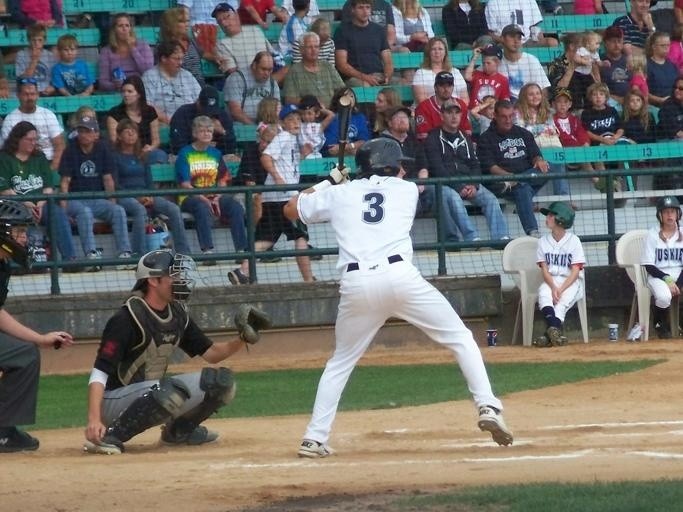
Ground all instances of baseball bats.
[338,96,352,171]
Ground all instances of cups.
[485,329,499,347]
[607,323,620,342]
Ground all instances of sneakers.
[65,248,322,286]
[537,326,567,346]
[0,426,40,454]
[81,436,125,455]
[479,405,513,446]
[160,426,219,447]
[296,439,335,458]
[654,322,671,338]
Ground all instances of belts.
[346,254,403,272]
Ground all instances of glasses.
[18,78,37,84]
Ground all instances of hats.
[200,87,221,115]
[211,3,235,18]
[72,115,99,130]
[386,25,572,119]
[278,95,317,120]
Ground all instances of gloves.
[327,162,352,185]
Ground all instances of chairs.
[615,229,683,342]
[0,0,683,235]
[502,236,589,347]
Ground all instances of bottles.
[256,122,276,140]
[111,67,127,80]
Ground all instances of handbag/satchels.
[27,234,47,267]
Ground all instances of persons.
[0,0,683,284]
[282,138,514,458]
[83,249,272,455]
[640,194,683,339]
[534,202,587,347]
[0,198,75,454]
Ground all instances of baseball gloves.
[234,303,273,343]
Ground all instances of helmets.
[135,248,197,314]
[0,200,36,271]
[539,200,574,229]
[355,138,415,178]
[656,196,682,221]
